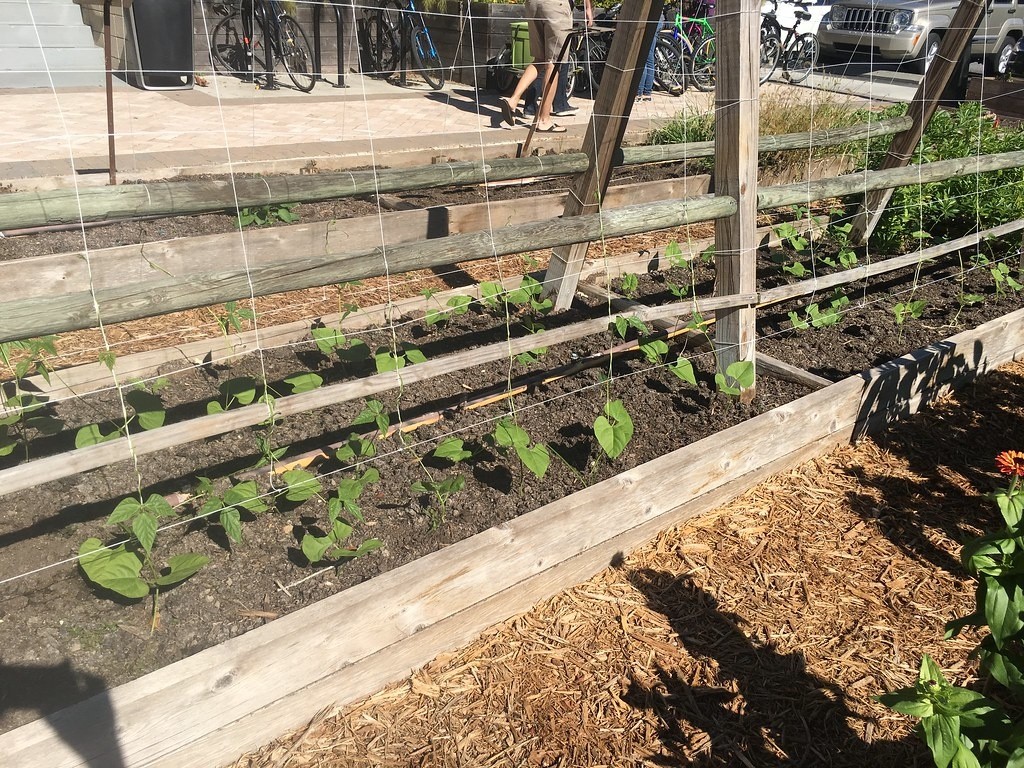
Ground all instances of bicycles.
[495,0,822,100]
[212,0,318,92]
[361,0,446,89]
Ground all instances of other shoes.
[523,103,538,114]
[635,95,644,102]
[556,106,580,115]
[643,96,651,101]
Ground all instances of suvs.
[815,0,1024,74]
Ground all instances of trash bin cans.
[511,23,534,74]
[942,42,972,105]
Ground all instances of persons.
[634,10,665,102]
[502,0,593,133]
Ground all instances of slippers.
[497,97,516,126]
[536,123,567,132]
